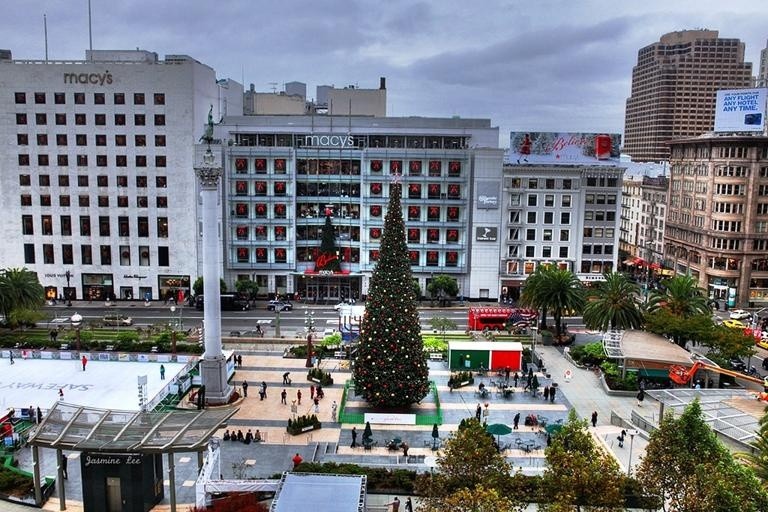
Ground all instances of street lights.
[66,270,72,306]
[169,305,176,327]
[626,429,639,476]
[304,311,316,367]
[530,327,538,363]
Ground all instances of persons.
[292,452,303,468]
[639,275,646,282]
[242,372,337,413]
[256,323,261,333]
[24,406,34,422]
[591,411,598,427]
[10,350,14,364]
[82,356,87,371]
[49,329,58,342]
[617,430,626,448]
[639,378,714,389]
[351,427,357,448]
[160,364,165,380]
[479,382,484,390]
[384,497,413,512]
[234,354,242,367]
[223,429,261,440]
[62,454,68,480]
[505,365,556,403]
[476,403,481,423]
[513,413,537,429]
[399,442,409,456]
[637,390,644,407]
[58,388,64,401]
[51,294,63,304]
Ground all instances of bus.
[468,306,540,331]
[196,294,250,311]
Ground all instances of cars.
[722,320,746,329]
[731,359,746,371]
[102,314,133,326]
[333,302,345,311]
[730,309,752,320]
[323,329,333,336]
[756,339,768,350]
[267,300,293,311]
